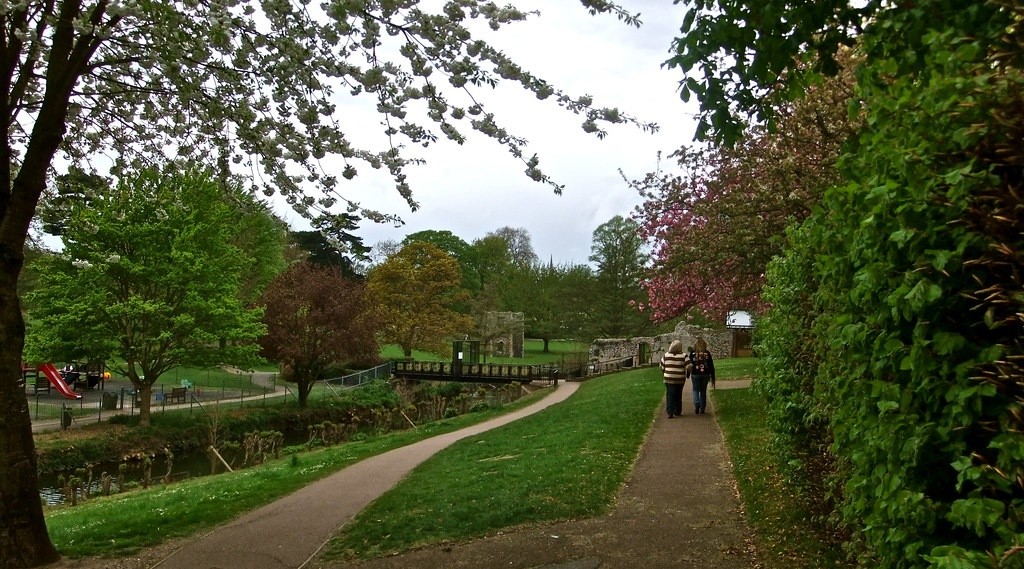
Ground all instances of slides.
[37,362,82,400]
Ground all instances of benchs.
[164,387,186,404]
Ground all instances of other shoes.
[668,413,673,419]
[700,410,704,414]
[695,404,700,414]
[674,411,681,416]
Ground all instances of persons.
[63,362,73,377]
[660,340,693,419]
[686,337,716,415]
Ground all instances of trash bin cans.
[102,392,118,410]
[63,406,72,427]
